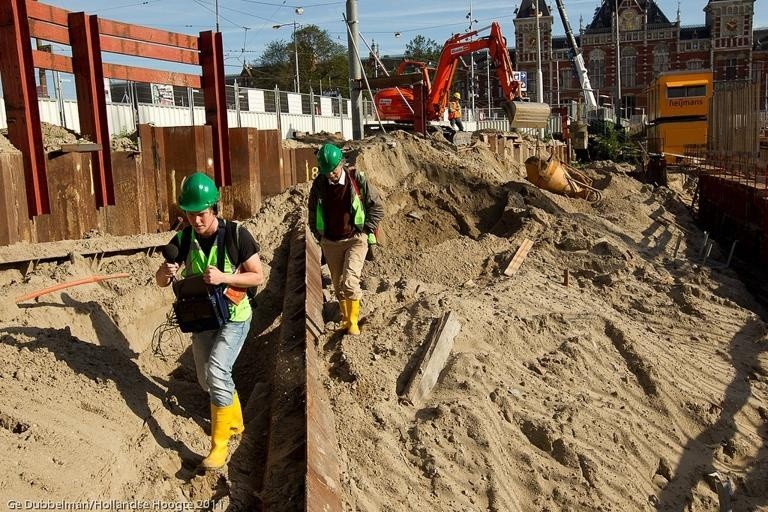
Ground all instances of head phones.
[214,201,220,213]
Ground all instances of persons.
[308,143,385,336]
[155,172,265,472]
[448,92,464,131]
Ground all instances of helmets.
[178,171,220,212]
[316,143,343,174]
[453,92,461,99]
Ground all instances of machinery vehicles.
[364,22,551,143]
[555,0,596,107]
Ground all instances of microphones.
[162,244,179,282]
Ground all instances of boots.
[200,402,234,471]
[228,389,246,439]
[338,300,349,329]
[346,299,361,335]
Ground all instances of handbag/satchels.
[172,273,232,334]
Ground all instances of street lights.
[371,40,378,78]
[272,20,300,95]
